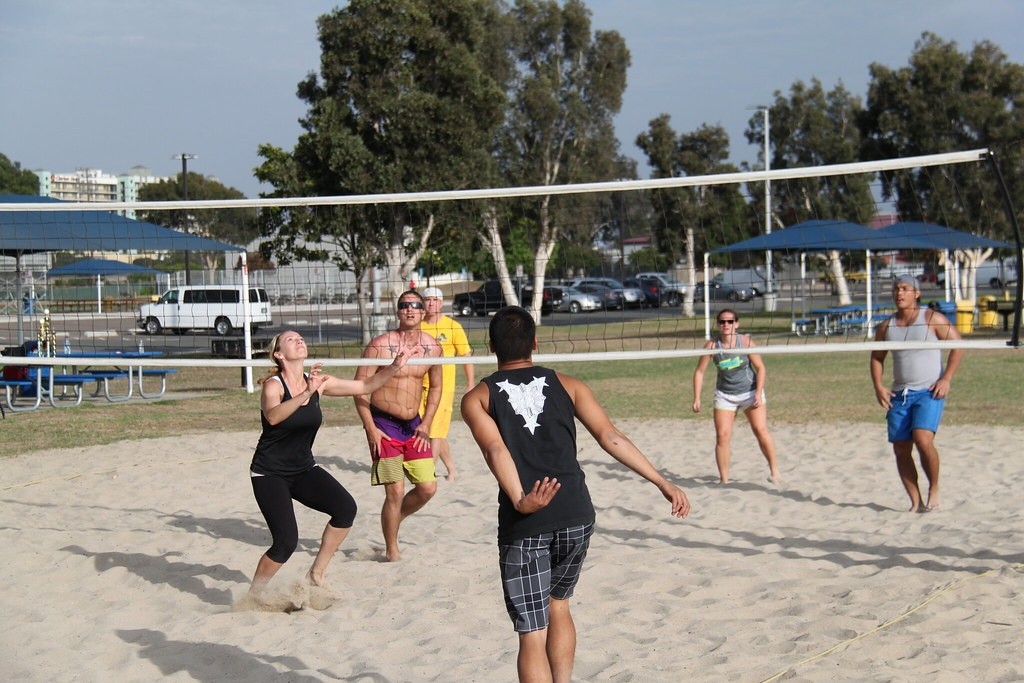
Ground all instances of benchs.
[795,315,891,337]
[0,369,179,412]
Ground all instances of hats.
[894,274,921,303]
[423,287,442,297]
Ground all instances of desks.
[27,350,162,399]
[808,303,893,335]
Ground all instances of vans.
[137,285,273,337]
[713,269,777,296]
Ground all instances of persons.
[693,309,779,483]
[354,291,443,562]
[870,274,965,513]
[460,304,690,683]
[410,280,415,291]
[418,287,474,482]
[247,331,419,594]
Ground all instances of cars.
[636,273,699,306]
[698,279,756,302]
[937,257,1018,290]
[573,285,626,307]
[624,278,661,308]
[818,264,867,282]
[543,286,602,313]
[563,278,645,309]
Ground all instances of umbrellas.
[710,219,934,332]
[870,222,1014,303]
[0,193,247,347]
[46,260,161,315]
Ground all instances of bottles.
[139,340,144,353]
[64,336,71,354]
[1004,287,1010,301]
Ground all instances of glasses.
[398,301,423,309]
[717,320,734,324]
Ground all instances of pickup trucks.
[452,280,563,317]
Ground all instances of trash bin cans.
[978,295,998,328]
[928,301,976,335]
[19,340,50,397]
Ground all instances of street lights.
[172,153,199,286]
[746,105,777,312]
